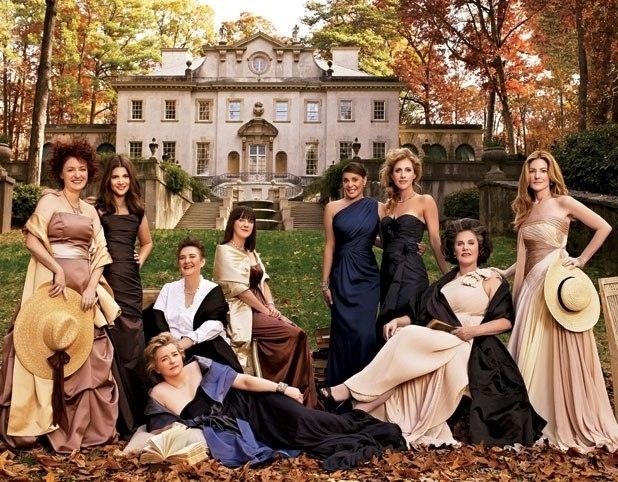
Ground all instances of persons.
[315,218,548,448]
[142,237,245,374]
[213,207,325,410]
[144,331,407,471]
[41,155,154,437]
[380,149,448,316]
[0,140,122,454]
[490,151,618,455]
[322,162,427,387]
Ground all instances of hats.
[543,259,601,332]
[13,285,95,380]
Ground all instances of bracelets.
[322,281,329,290]
[275,382,289,394]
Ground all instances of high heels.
[318,386,350,411]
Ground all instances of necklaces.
[61,190,83,216]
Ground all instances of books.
[140,424,208,467]
